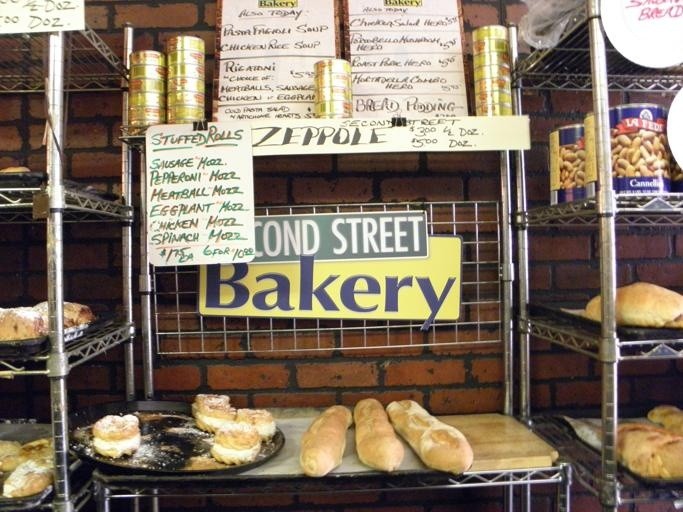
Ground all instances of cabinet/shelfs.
[134,116,561,510]
[0,22,134,512]
[506,0,682,512]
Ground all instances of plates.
[65,399,285,478]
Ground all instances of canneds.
[549,103,683,206]
[166,35,205,124]
[128,50,166,135]
[472,25,512,116]
[313,59,353,118]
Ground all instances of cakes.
[93,414,140,459]
[191,393,275,466]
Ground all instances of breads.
[618,405,683,483]
[581,281,683,328]
[0,301,96,341]
[0,438,54,498]
[0,166,30,172]
[300,398,474,478]
